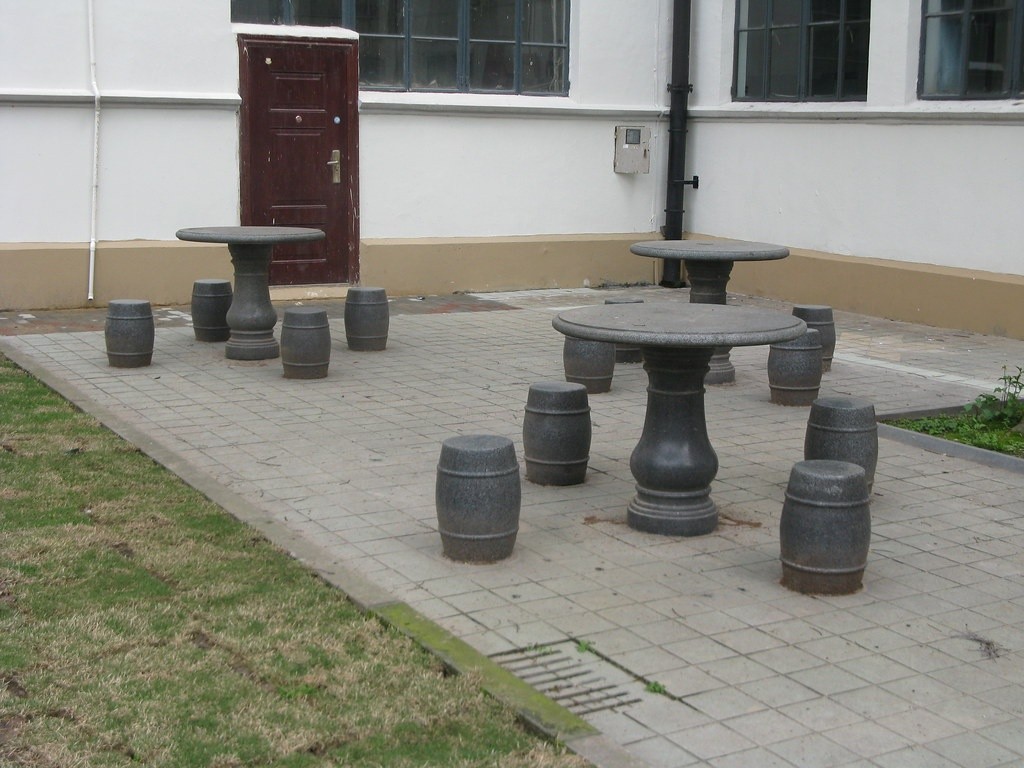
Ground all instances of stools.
[344,287,389,350]
[281,307,331,379]
[779,460,871,596]
[522,382,591,486]
[435,435,521,562]
[564,336,617,393]
[105,299,154,367]
[768,329,823,406]
[191,279,233,342]
[605,299,644,363]
[803,397,878,493]
[792,304,836,372]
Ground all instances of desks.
[176,226,326,360]
[630,240,790,384]
[551,303,807,537]
